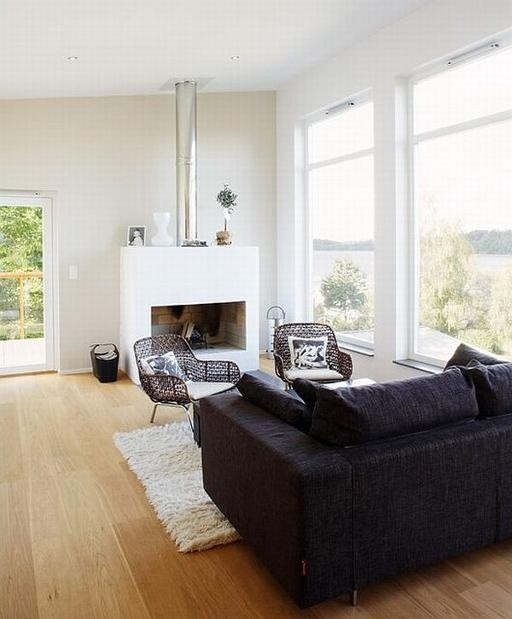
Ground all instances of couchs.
[200,343,512,610]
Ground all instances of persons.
[131,229,143,245]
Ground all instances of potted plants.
[215,182,239,246]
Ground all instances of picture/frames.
[127,226,148,246]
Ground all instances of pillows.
[311,365,479,447]
[287,335,329,370]
[235,372,307,430]
[290,378,337,411]
[140,352,194,392]
[469,360,512,416]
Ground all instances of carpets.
[110,420,249,552]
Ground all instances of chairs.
[132,335,243,438]
[270,323,353,390]
